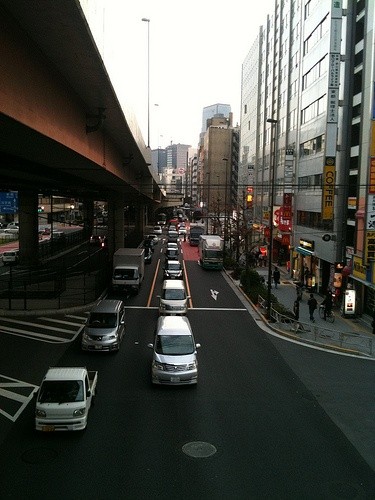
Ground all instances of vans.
[147,315,202,386]
[156,279,191,316]
[79,299,127,353]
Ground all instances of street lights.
[265,119,279,319]
[206,172,211,235]
[141,17,151,148]
[222,158,229,243]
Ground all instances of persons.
[320,294,333,320]
[277,252,282,266]
[305,267,311,288]
[299,268,305,287]
[296,283,304,303]
[293,296,301,321]
[325,287,334,300]
[273,267,280,289]
[307,293,318,323]
[251,250,267,267]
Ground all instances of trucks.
[111,247,146,295]
[33,366,99,433]
[188,222,206,246]
[197,235,224,271]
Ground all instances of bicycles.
[317,303,336,324]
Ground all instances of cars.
[162,260,184,280]
[89,234,106,245]
[38,206,44,214]
[0,219,7,229]
[6,221,20,234]
[1,250,18,266]
[141,207,188,264]
